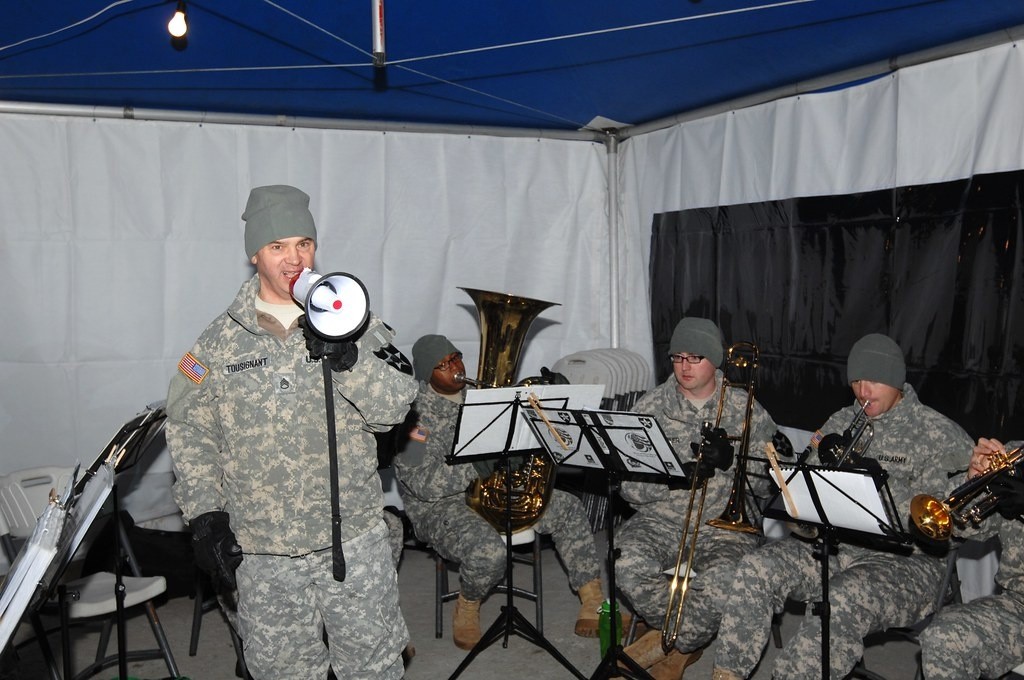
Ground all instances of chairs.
[551,347,649,535]
[847,544,962,680]
[0,466,218,680]
[435,526,543,638]
[623,562,697,647]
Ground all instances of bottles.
[597,598,623,659]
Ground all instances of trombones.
[658,341,764,655]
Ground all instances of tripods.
[446,390,589,680]
[522,407,692,680]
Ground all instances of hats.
[846,333,906,390]
[413,334,461,385]
[667,317,723,369]
[242,185,318,260]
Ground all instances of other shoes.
[402,644,415,658]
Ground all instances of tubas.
[451,283,559,536]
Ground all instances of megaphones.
[289,267,370,339]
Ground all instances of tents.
[0,0,1024,574]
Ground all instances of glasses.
[434,353,462,371]
[668,355,705,363]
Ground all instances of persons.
[713,334,975,680]
[613,316,797,680]
[164,185,419,680]
[393,334,646,649]
[918,438,1024,680]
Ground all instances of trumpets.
[909,444,1023,541]
[784,400,876,540]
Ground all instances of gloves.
[691,427,734,472]
[668,461,715,490]
[188,511,242,606]
[540,367,570,385]
[987,461,1024,520]
[298,314,358,372]
[818,429,853,464]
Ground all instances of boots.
[608,629,676,680]
[648,647,703,680]
[453,593,482,651]
[575,578,648,638]
[711,668,744,680]
